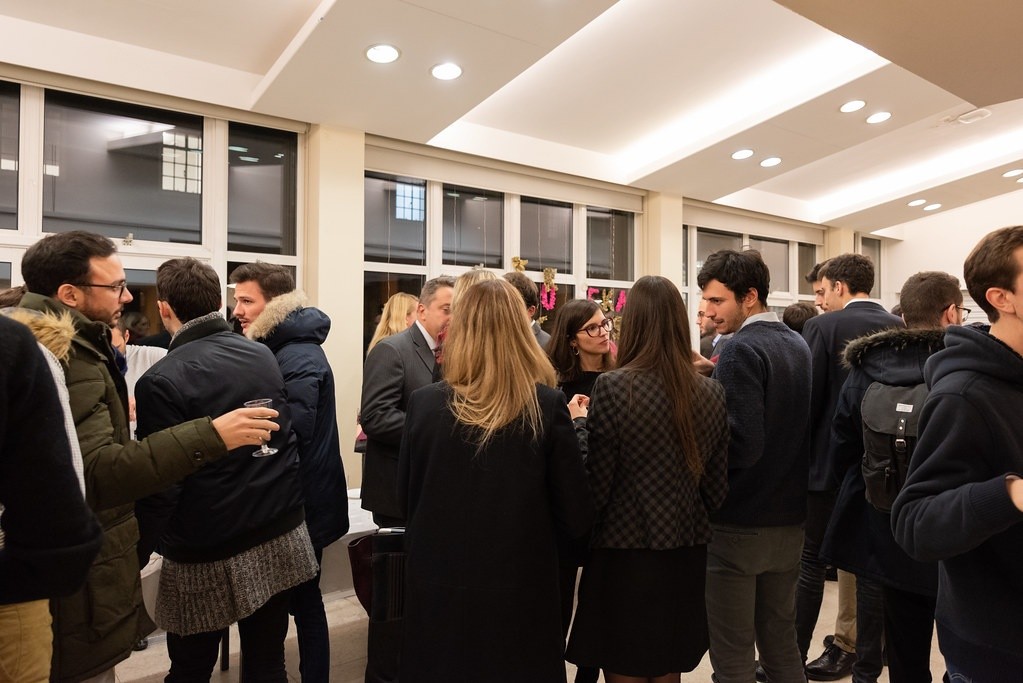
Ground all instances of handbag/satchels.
[348,525,408,620]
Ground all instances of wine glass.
[244,398,279,457]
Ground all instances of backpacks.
[861,381,930,513]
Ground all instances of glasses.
[571,317,613,337]
[943,305,971,322]
[56,282,127,299]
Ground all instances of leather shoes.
[806,643,857,680]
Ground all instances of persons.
[397,278,584,683]
[756,253,906,683]
[0,226,350,683]
[892,226,1023,683]
[697,249,813,683]
[360,257,835,683]
[569,278,729,683]
[820,272,991,683]
